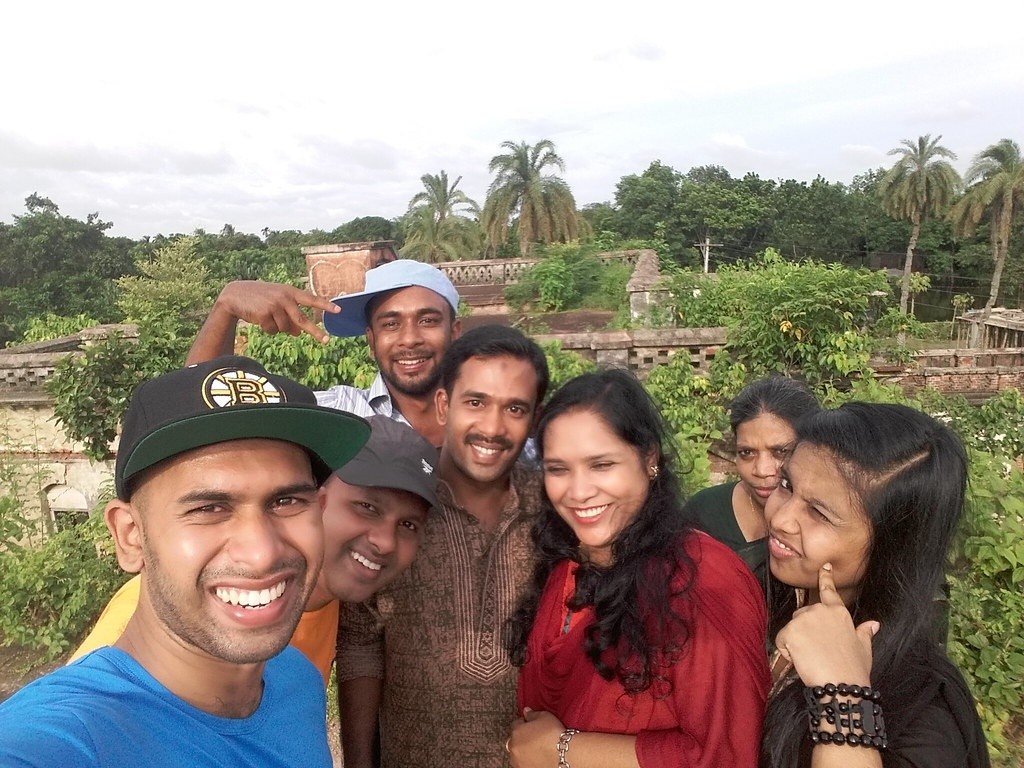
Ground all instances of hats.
[323,259,461,338]
[115,356,373,502]
[335,414,440,508]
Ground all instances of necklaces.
[750,493,755,512]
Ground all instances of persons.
[336,326,549,768]
[503,370,773,768]
[0,355,372,768]
[183,259,462,456]
[683,379,819,587]
[757,402,991,768]
[65,414,438,693]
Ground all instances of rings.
[505,737,511,753]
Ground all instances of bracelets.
[557,729,579,768]
[807,684,888,750]
